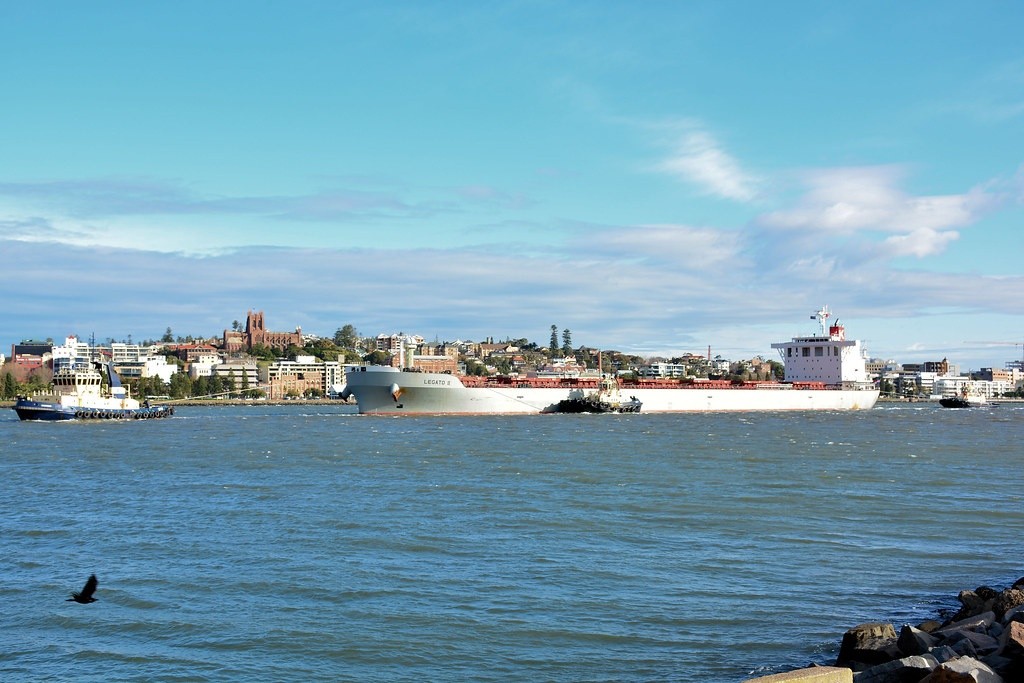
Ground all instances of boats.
[10,352,175,422]
[564,371,643,414]
[938,397,974,410]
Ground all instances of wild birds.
[65,574,99,604]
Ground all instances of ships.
[346,304,881,415]
